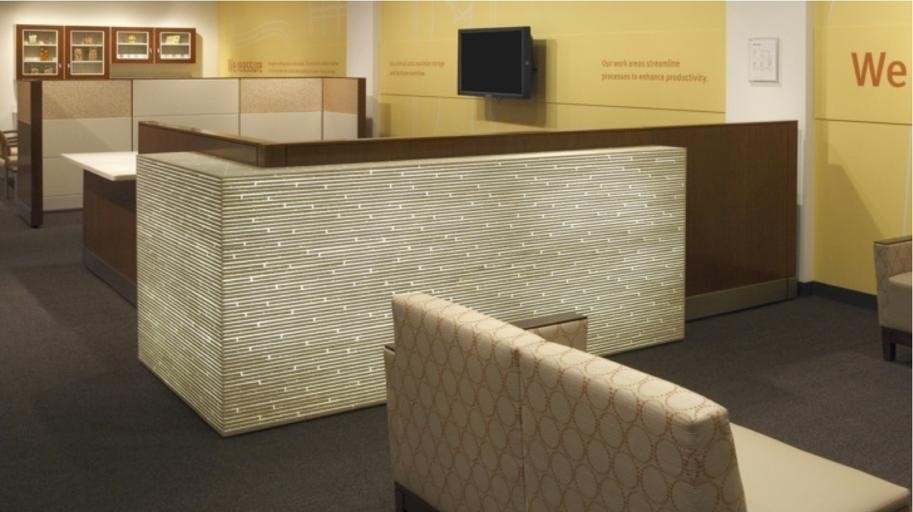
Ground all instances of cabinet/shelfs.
[15,24,197,81]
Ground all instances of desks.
[57,152,135,310]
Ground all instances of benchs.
[383,289,913,512]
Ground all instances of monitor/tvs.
[457,26,533,98]
